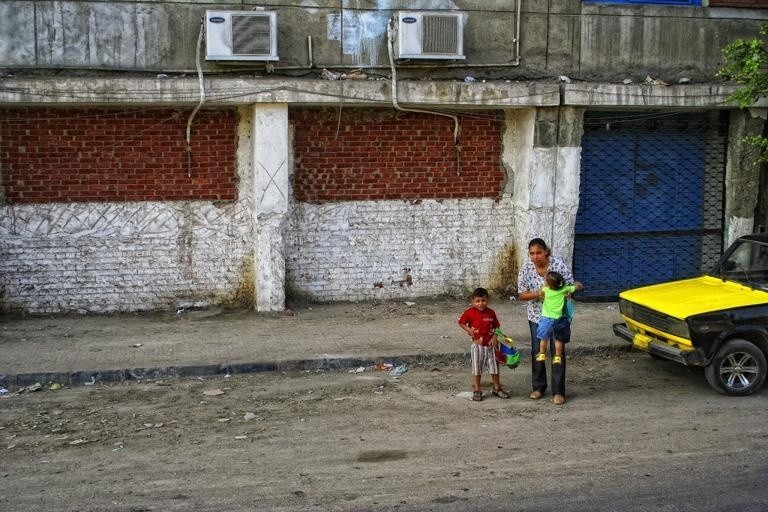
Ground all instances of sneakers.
[472,390,482,401]
[536,353,546,362]
[552,355,562,365]
[492,386,510,398]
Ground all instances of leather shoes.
[530,391,542,399]
[554,394,564,405]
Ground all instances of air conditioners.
[203,10,280,61]
[393,10,465,59]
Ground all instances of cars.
[613,233,768,396]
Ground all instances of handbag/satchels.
[494,329,520,369]
[553,316,571,342]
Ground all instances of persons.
[458,287,510,401]
[535,271,582,364]
[516,239,575,404]
